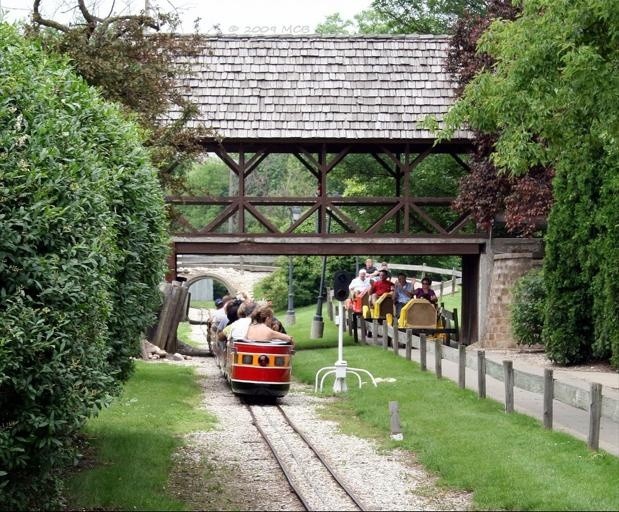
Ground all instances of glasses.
[422,283,428,286]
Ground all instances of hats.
[215,299,224,306]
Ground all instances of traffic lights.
[333,271,351,301]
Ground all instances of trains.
[344,288,449,350]
[206,313,296,403]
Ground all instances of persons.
[348,259,412,319]
[411,277,438,310]
[207,292,294,345]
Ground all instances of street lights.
[285,206,300,326]
[309,191,342,338]
